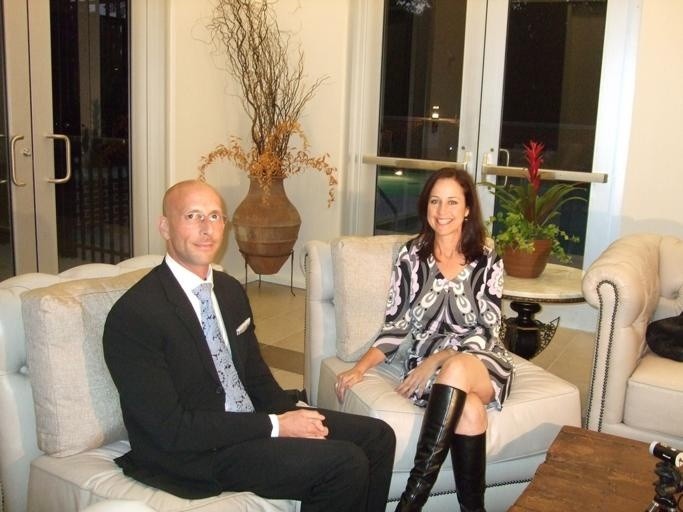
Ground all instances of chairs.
[0,252,319,510]
[578,233,683,452]
[298,233,581,510]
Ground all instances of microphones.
[648,440,683,469]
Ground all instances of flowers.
[477,137,592,266]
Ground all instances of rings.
[335,383,339,388]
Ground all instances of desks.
[495,257,588,362]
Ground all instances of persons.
[103,179,396,512]
[335,167,516,512]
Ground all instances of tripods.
[645,495,677,511]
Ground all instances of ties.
[192,284,255,412]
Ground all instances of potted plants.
[194,0,340,272]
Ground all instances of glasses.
[184,211,228,222]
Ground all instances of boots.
[396,382,487,512]
